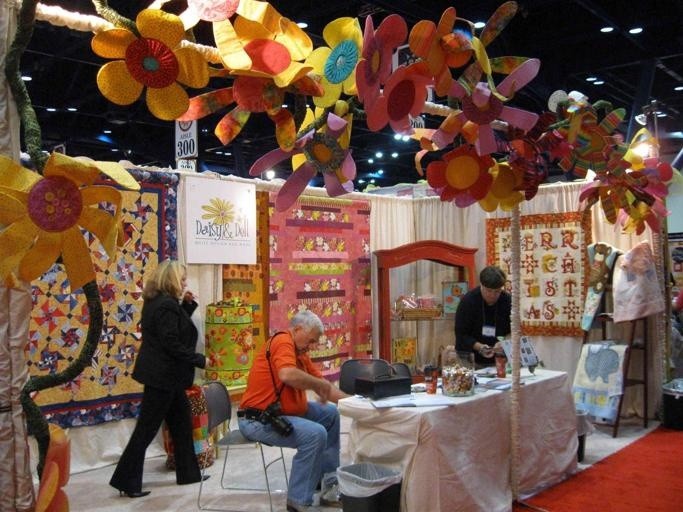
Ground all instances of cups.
[441,350,473,396]
[494,350,507,379]
[424,365,438,395]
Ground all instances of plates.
[410,384,427,392]
[474,366,512,391]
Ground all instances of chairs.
[390,362,413,388]
[338,358,394,395]
[197,381,290,512]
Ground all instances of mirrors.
[667,233,683,379]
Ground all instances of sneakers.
[286,499,322,512]
[319,496,343,509]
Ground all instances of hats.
[480,266,505,289]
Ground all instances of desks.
[337,362,590,512]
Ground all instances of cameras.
[260,401,293,437]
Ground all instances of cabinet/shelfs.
[372,240,478,364]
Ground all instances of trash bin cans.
[335,462,403,512]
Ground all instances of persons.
[237,309,353,512]
[454,266,512,370]
[671,286,683,311]
[106,259,212,498]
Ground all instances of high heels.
[119,488,152,498]
